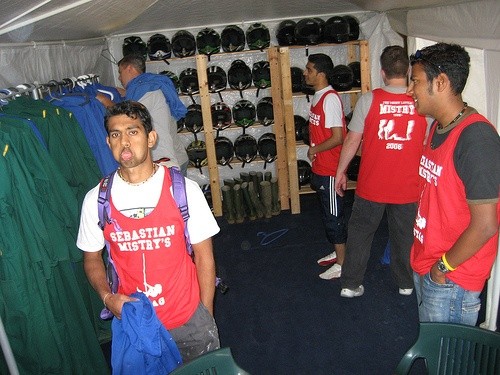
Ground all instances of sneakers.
[317,251,337,266]
[340,285,364,298]
[319,263,341,280]
[399,287,413,295]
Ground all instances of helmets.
[147,34,172,61]
[196,27,221,56]
[300,76,315,95]
[200,184,213,209]
[276,15,359,47]
[302,120,310,145]
[214,137,234,166]
[123,36,147,61]
[297,159,311,186]
[246,23,270,50]
[291,67,304,93]
[186,141,208,168]
[284,115,305,140]
[234,134,258,163]
[221,25,245,53]
[171,30,196,58]
[347,155,361,181]
[158,60,274,133]
[258,133,278,163]
[347,61,361,88]
[331,65,354,92]
[345,116,350,133]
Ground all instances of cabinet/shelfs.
[146,39,372,217]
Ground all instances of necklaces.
[436,102,467,130]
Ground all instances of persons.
[405,41,500,375]
[333,45,431,296]
[303,53,346,278]
[76,55,220,367]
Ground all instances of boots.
[221,170,280,225]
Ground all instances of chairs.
[396,322,500,375]
[169,346,250,375]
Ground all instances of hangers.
[0,74,114,106]
[101,41,118,65]
[257,229,289,246]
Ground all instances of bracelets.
[442,252,457,271]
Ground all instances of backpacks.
[100,165,194,320]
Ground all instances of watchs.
[437,257,451,273]
